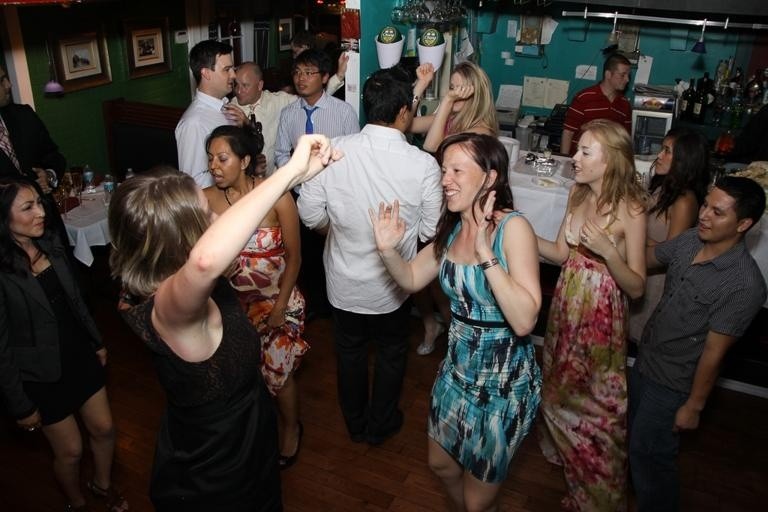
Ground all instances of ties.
[247,103,260,121]
[0,113,23,176]
[303,105,320,134]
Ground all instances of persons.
[560,54,632,156]
[106,132,337,512]
[174,39,265,193]
[0,64,67,252]
[366,133,543,512]
[626,129,707,348]
[629,176,768,510]
[230,50,349,181]
[185,125,306,470]
[298,66,445,447]
[0,176,131,512]
[275,49,361,320]
[536,119,648,512]
[401,62,501,356]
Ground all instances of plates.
[532,177,563,189]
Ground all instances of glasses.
[291,70,321,77]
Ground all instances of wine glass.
[49,174,84,219]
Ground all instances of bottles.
[83,164,96,193]
[125,166,135,179]
[103,173,116,206]
[250,113,257,130]
[680,57,768,122]
[639,117,650,154]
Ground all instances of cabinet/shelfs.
[628,107,675,165]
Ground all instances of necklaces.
[224,176,255,207]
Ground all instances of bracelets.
[479,257,499,271]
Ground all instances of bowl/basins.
[645,105,661,111]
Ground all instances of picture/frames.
[45,16,113,94]
[118,14,174,83]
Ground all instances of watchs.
[46,170,59,190]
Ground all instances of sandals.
[87,478,130,512]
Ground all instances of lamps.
[691,18,709,55]
[606,10,621,49]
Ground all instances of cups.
[516,125,549,151]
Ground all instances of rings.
[28,427,35,432]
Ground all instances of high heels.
[417,320,447,356]
[278,420,304,469]
